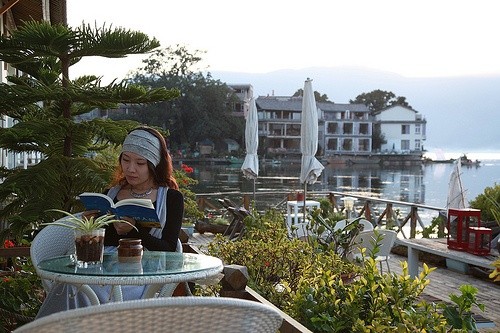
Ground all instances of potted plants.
[40,208,138,268]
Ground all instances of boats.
[455,155,473,166]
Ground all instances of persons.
[36,125,184,322]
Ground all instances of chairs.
[217,197,254,242]
[30,211,183,305]
[14,297,283,333]
[334,218,398,275]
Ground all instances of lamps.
[341,196,358,211]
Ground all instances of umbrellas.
[240,97,259,216]
[301,77,325,223]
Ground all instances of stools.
[447,208,492,255]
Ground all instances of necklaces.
[130,184,153,197]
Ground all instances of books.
[79,193,162,228]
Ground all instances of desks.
[36,251,223,308]
[287,200,320,231]
[399,238,500,280]
